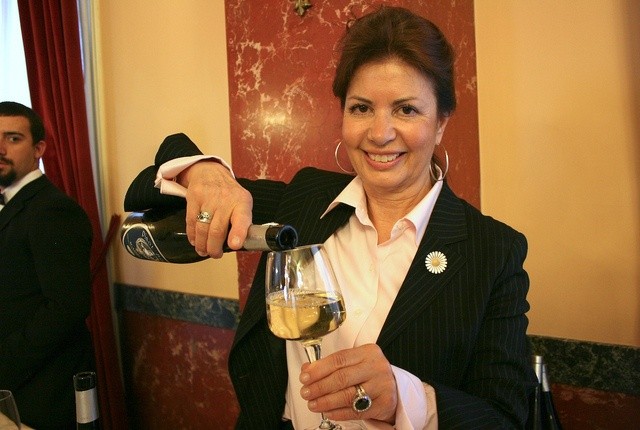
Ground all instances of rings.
[197,212,211,225]
[352,384,372,413]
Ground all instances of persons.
[1,101,97,430]
[124,7,539,429]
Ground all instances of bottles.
[121,206,297,266]
[74,370,99,428]
[528,354,560,429]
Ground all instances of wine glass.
[0,389,21,429]
[266,246,346,430]
